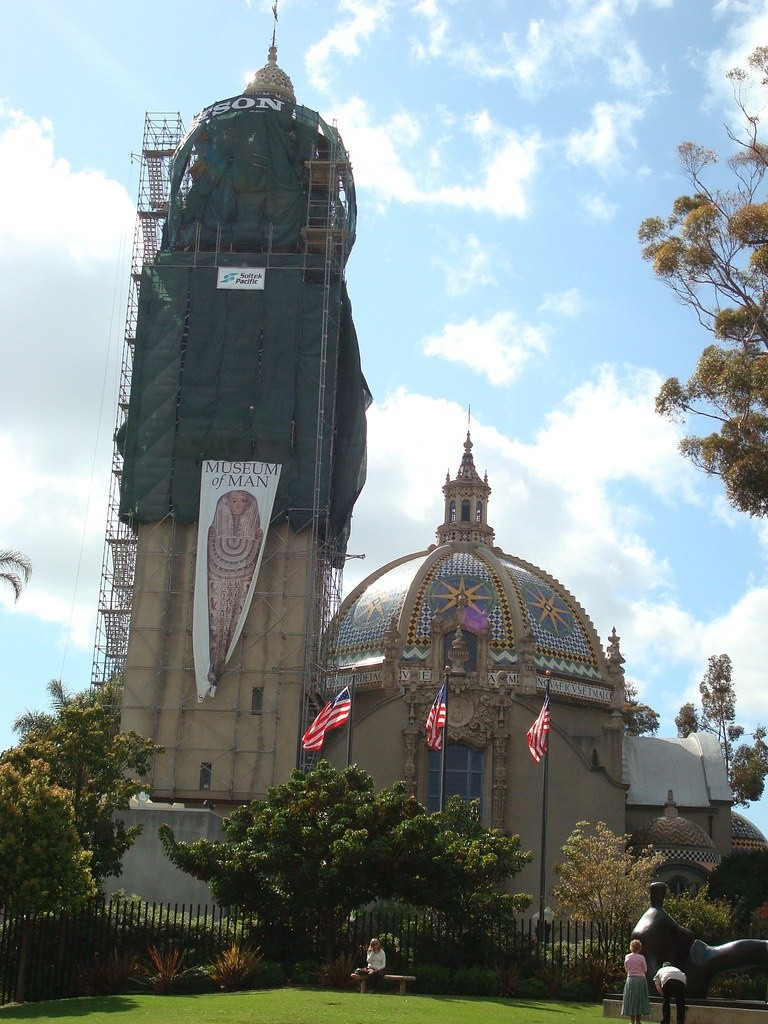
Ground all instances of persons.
[652,961,688,1024]
[354,938,386,994]
[621,939,651,1024]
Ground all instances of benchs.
[351,973,416,996]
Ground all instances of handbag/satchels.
[355,968,369,978]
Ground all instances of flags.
[301,686,350,752]
[425,684,447,752]
[527,694,550,765]
